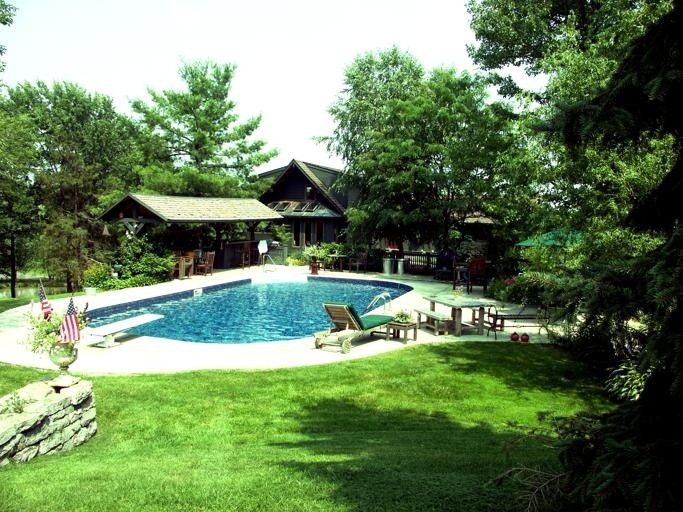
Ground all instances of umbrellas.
[514,229,582,277]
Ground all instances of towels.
[258,240,268,256]
[266,238,272,245]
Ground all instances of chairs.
[315,302,397,352]
[234,241,251,268]
[170,250,216,279]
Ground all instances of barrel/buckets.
[383,256,393,274]
[394,259,411,274]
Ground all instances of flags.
[40,284,51,322]
[58,298,80,344]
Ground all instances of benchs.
[472,307,504,331]
[486,304,551,340]
[413,308,453,335]
[347,263,363,272]
[311,261,325,271]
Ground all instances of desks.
[422,293,496,337]
[386,322,417,344]
[326,254,347,271]
